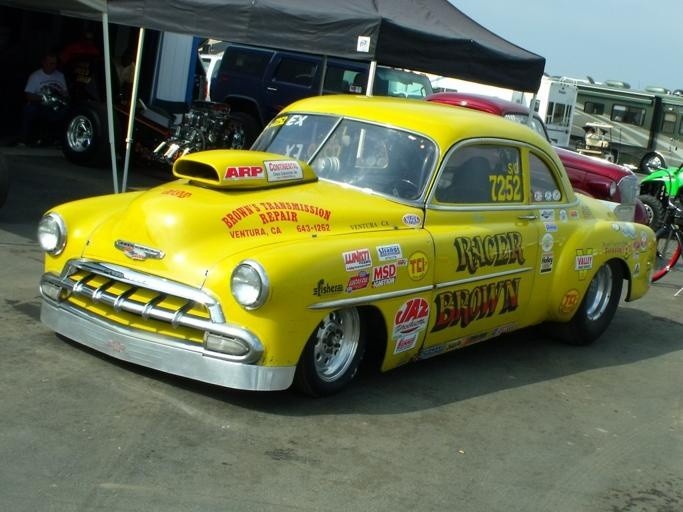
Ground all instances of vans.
[210,45,434,150]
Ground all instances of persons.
[66,47,100,97]
[14,46,73,150]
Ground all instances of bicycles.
[651,168,683,282]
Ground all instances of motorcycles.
[48,87,245,176]
